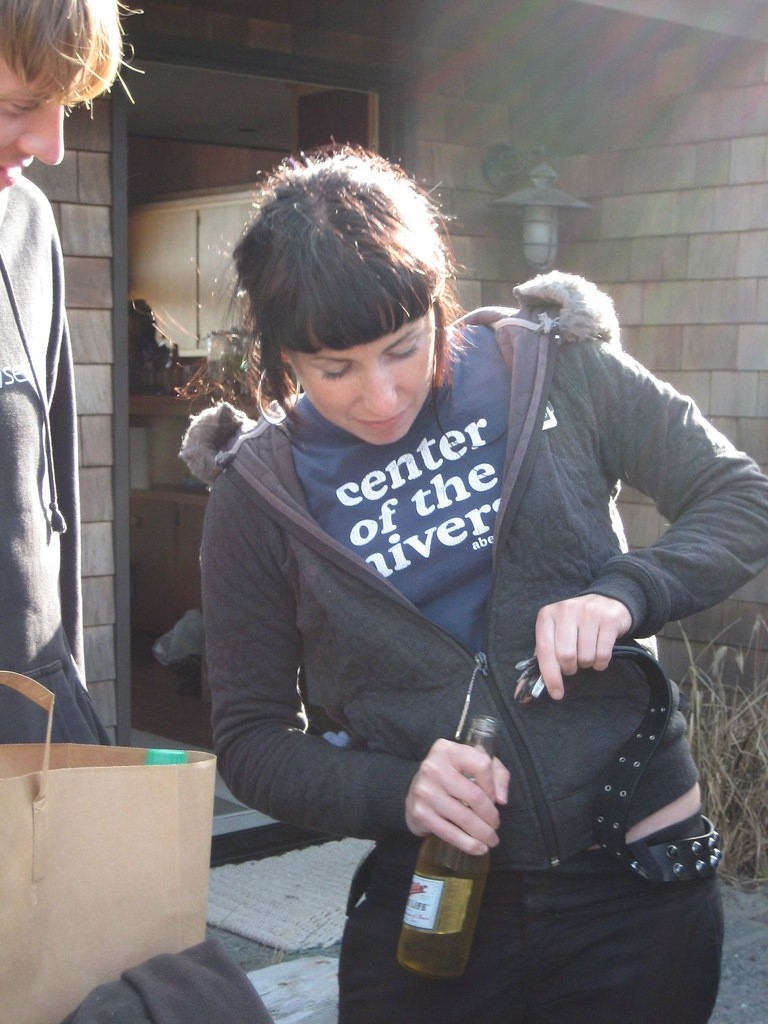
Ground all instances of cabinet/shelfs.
[125,183,272,357]
[130,490,208,653]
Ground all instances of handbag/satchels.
[0,670,217,1024]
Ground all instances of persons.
[0,0,126,747]
[179,149,768,1024]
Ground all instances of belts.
[509,646,723,886]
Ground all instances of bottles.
[396,714,503,981]
